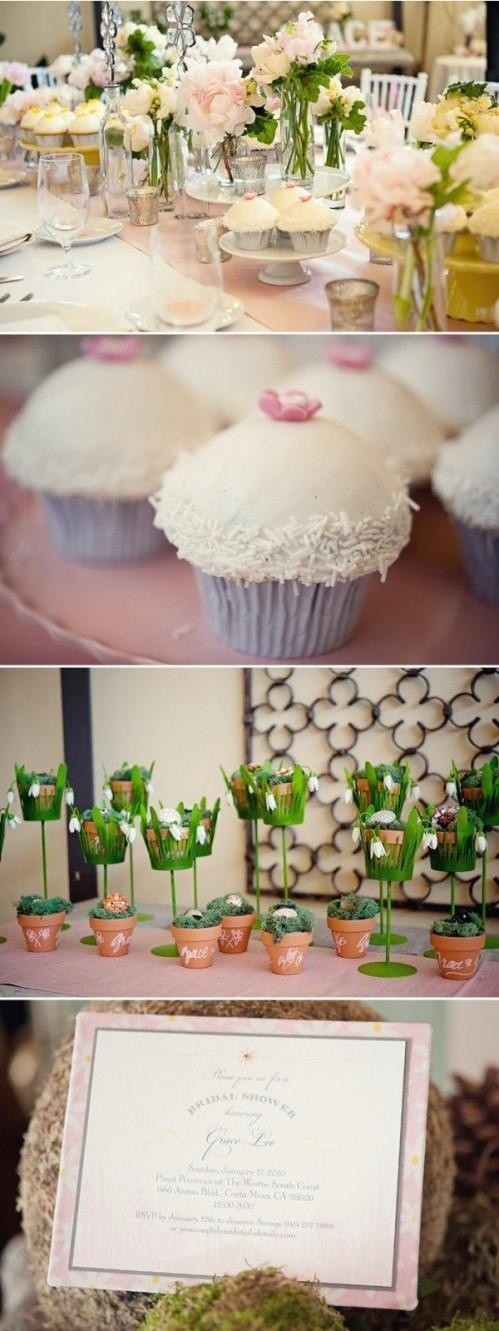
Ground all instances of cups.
[127,185,162,228]
[192,218,232,266]
[230,154,267,192]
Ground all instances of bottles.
[148,219,225,326]
[168,85,204,219]
[327,280,380,333]
[100,83,134,194]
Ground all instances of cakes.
[0,335,499,658]
[19,99,106,147]
[222,182,338,254]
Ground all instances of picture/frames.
[47,1012,432,1310]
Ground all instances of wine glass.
[36,150,93,278]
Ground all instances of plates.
[0,218,35,258]
[0,169,24,190]
[22,215,122,243]
[133,287,247,333]
